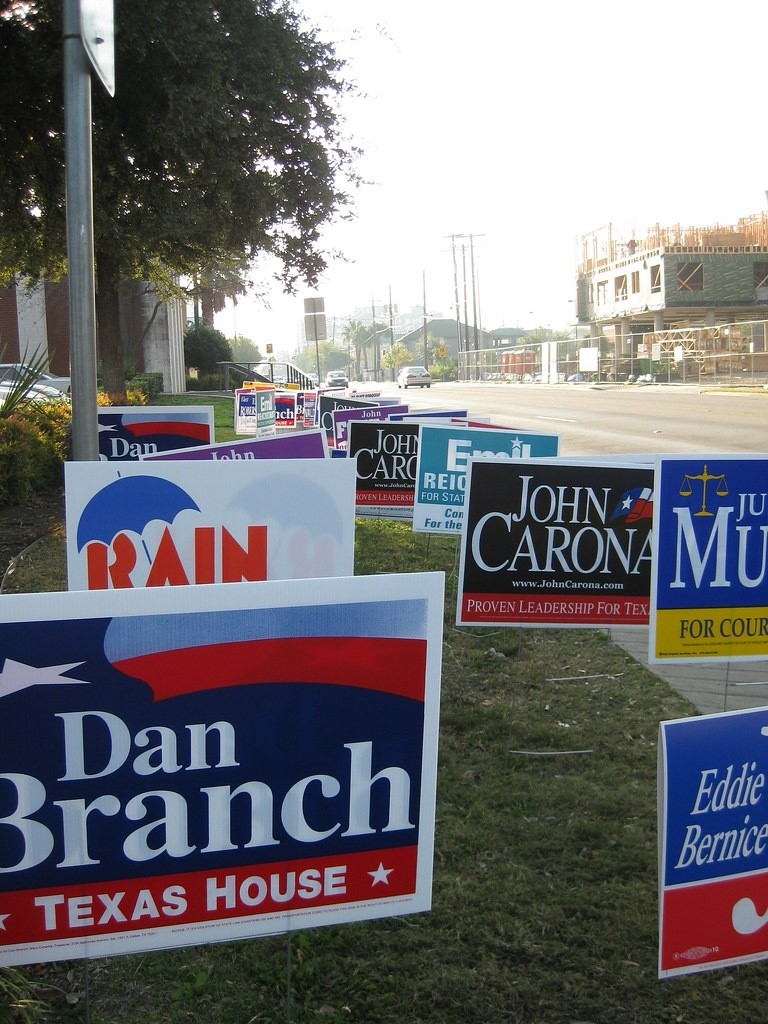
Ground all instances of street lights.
[567,300,579,373]
[627,332,633,375]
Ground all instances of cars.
[567,373,583,381]
[606,359,640,381]
[645,373,666,383]
[0,364,71,396]
[0,386,49,410]
[398,367,431,389]
[325,371,349,388]
[0,380,66,400]
[628,366,681,383]
